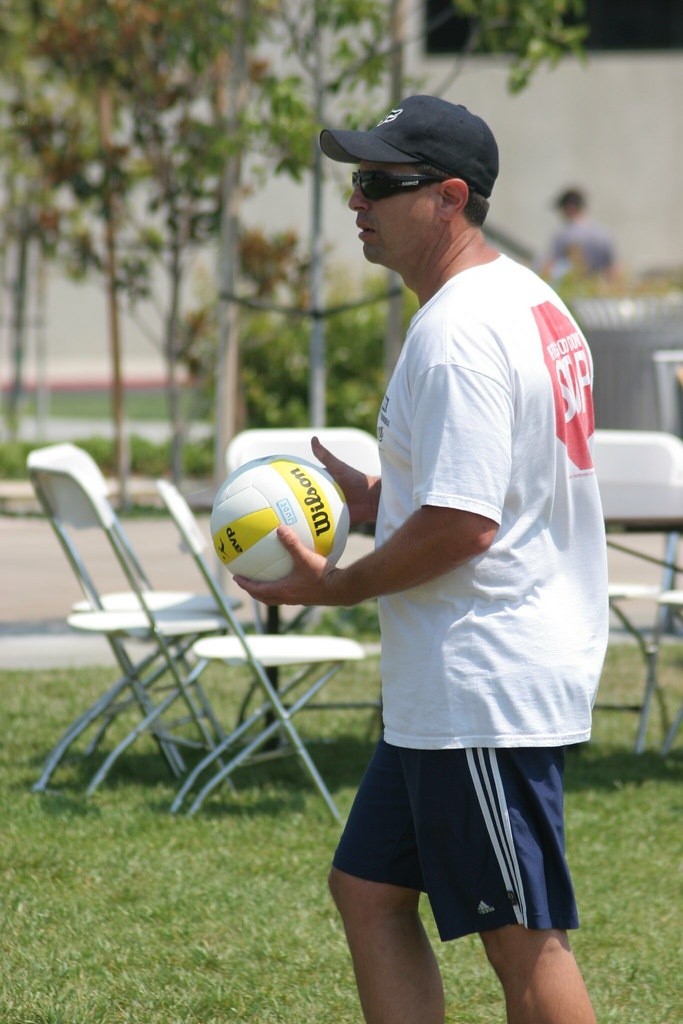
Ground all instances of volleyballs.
[208,453,352,584]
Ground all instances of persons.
[547,185,619,276]
[229,93,607,1024]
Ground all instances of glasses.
[351,170,475,200]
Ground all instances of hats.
[320,95,500,199]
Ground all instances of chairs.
[584,428,683,755]
[25,427,384,824]
